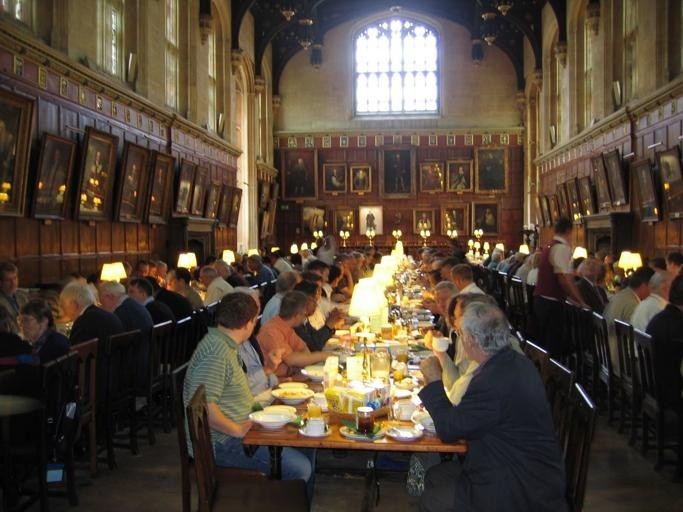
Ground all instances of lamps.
[177,228,643,277]
[280,0,318,50]
[481,0,512,47]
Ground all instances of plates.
[249,381,314,431]
[387,254,431,397]
[338,411,435,442]
[300,366,328,382]
[295,427,332,439]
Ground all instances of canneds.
[356,407,374,435]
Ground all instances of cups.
[311,390,326,411]
[432,336,448,352]
[393,399,415,420]
[369,351,392,379]
[305,418,326,433]
[356,406,376,433]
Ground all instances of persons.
[417,248,571,510]
[468,247,682,413]
[532,216,593,356]
[292,247,392,353]
[311,227,337,271]
[1,250,306,510]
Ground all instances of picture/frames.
[538,143,683,229]
[258,133,511,242]
[0,85,243,229]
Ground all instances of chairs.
[461,261,682,511]
[0,263,306,512]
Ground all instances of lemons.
[393,371,404,381]
[398,355,408,363]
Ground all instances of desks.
[244,279,467,511]
